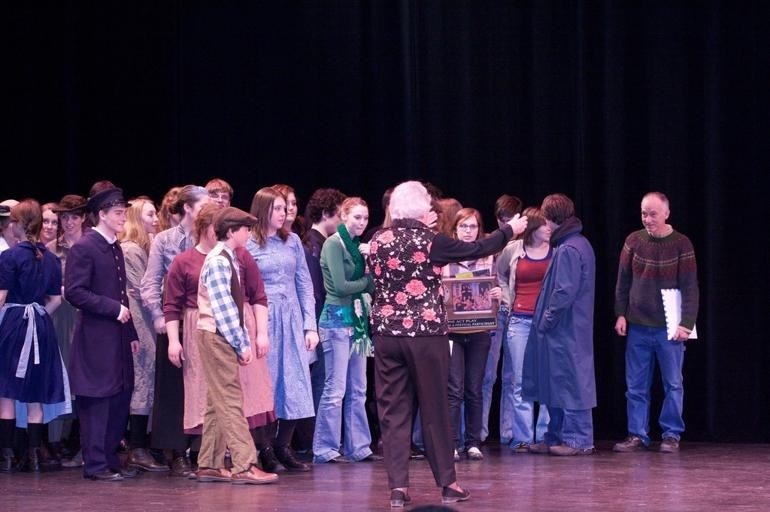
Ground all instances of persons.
[2,177,595,485]
[607,189,704,456]
[520,190,597,458]
[196,204,280,487]
[361,180,529,512]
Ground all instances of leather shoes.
[169,457,197,478]
[16,447,41,473]
[660,438,680,453]
[197,469,232,482]
[514,441,530,453]
[549,443,593,456]
[273,445,312,471]
[259,446,288,473]
[363,452,384,461]
[61,449,85,468]
[328,455,352,464]
[112,465,140,478]
[127,448,170,472]
[529,442,550,453]
[390,490,411,507]
[231,465,279,484]
[0,456,16,472]
[612,433,648,452]
[410,444,425,460]
[442,486,471,503]
[92,469,124,483]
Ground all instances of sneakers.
[453,449,460,461]
[466,447,483,460]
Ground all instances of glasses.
[459,223,478,230]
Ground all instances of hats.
[214,206,259,232]
[0,199,18,217]
[51,194,87,212]
[86,188,132,213]
